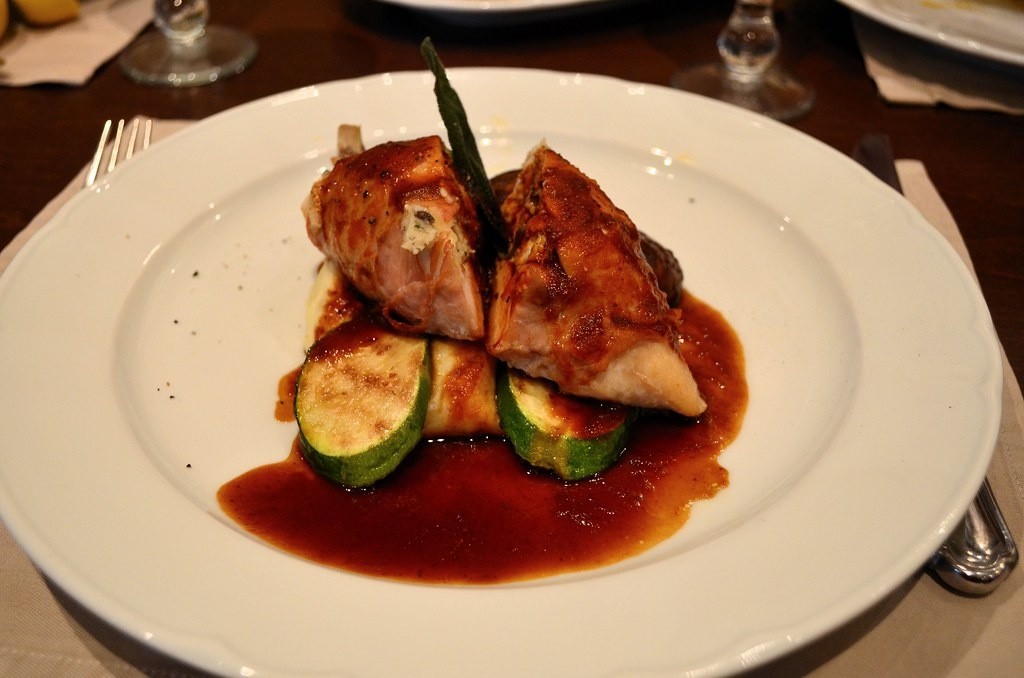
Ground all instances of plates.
[835,1,1022,67]
[0,66,1005,678]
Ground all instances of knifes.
[848,140,1017,598]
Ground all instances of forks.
[83,118,155,188]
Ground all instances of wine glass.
[669,0,814,120]
[110,0,258,87]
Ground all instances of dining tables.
[1,0,1024,678]
